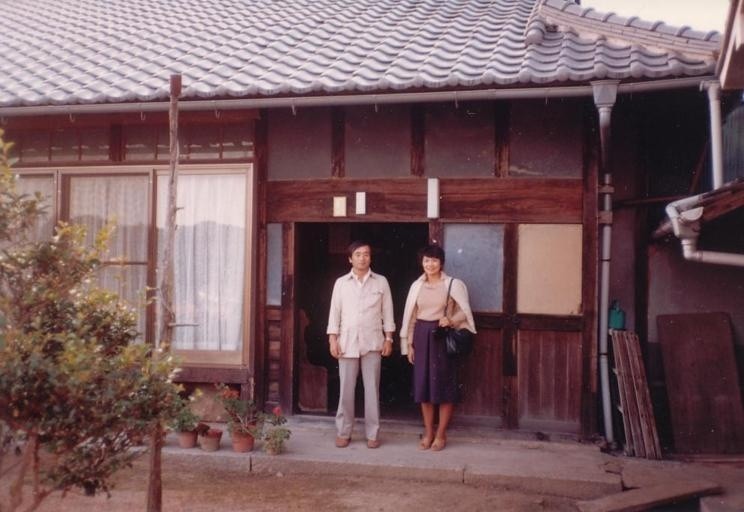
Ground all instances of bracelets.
[384,336,394,343]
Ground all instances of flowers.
[172,381,293,456]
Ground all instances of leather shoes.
[336,435,351,447]
[367,439,382,448]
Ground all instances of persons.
[324,239,396,449]
[398,245,476,451]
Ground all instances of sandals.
[420,431,447,451]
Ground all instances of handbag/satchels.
[445,329,474,361]
